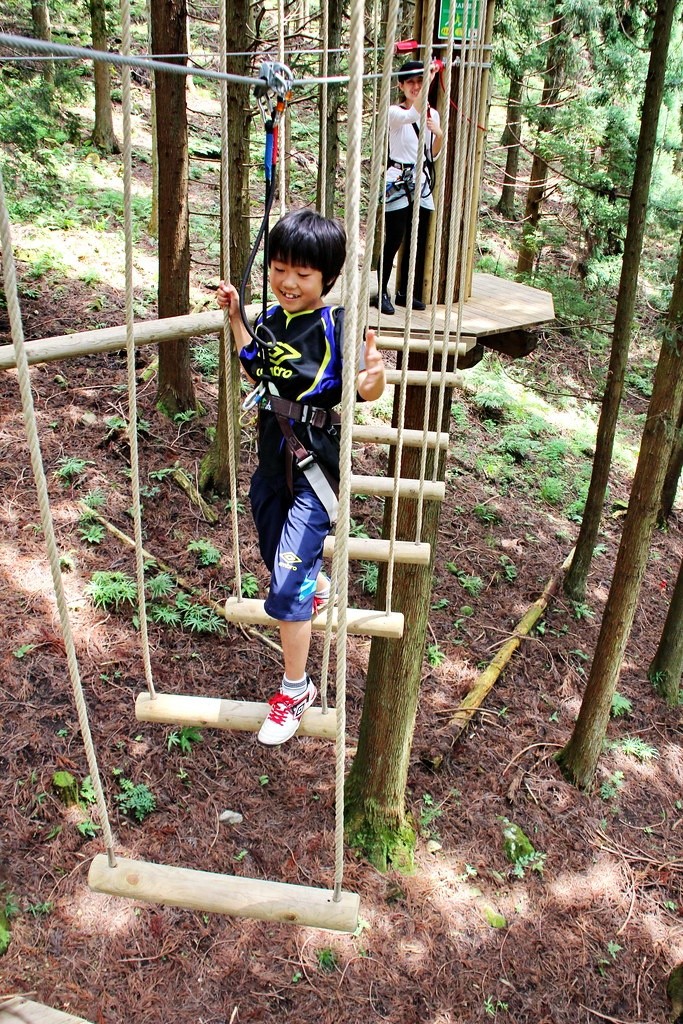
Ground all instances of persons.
[215,207,386,748]
[377,61,446,316]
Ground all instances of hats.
[398,62,424,82]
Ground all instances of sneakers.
[375,294,395,314]
[311,577,339,617]
[395,290,426,310]
[257,677,318,746]
